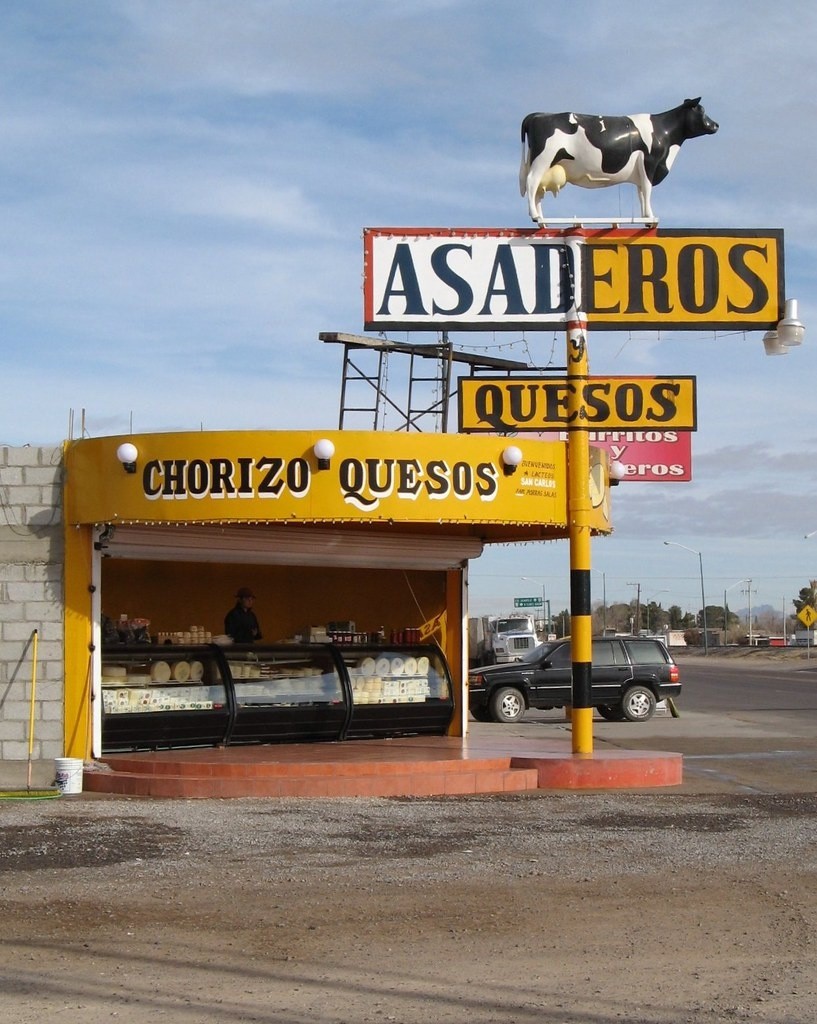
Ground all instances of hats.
[235,587,257,599]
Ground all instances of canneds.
[391,627,420,643]
[327,631,368,644]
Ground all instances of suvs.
[469,637,682,723]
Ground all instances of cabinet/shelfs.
[101,642,233,752]
[325,643,456,741]
[213,641,351,746]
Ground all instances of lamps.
[116,443,139,474]
[313,439,335,470]
[502,446,522,475]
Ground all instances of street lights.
[520,577,547,644]
[663,540,708,657]
[627,581,640,636]
[647,589,669,636]
[724,578,752,647]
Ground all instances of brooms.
[0,628,62,800]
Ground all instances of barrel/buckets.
[55,757,84,794]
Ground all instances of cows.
[519,96,721,227]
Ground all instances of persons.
[224,588,262,644]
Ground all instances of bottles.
[380,626,384,636]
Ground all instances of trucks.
[468,614,543,668]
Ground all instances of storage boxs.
[302,636,329,643]
[304,624,326,636]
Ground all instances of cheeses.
[101,657,430,704]
